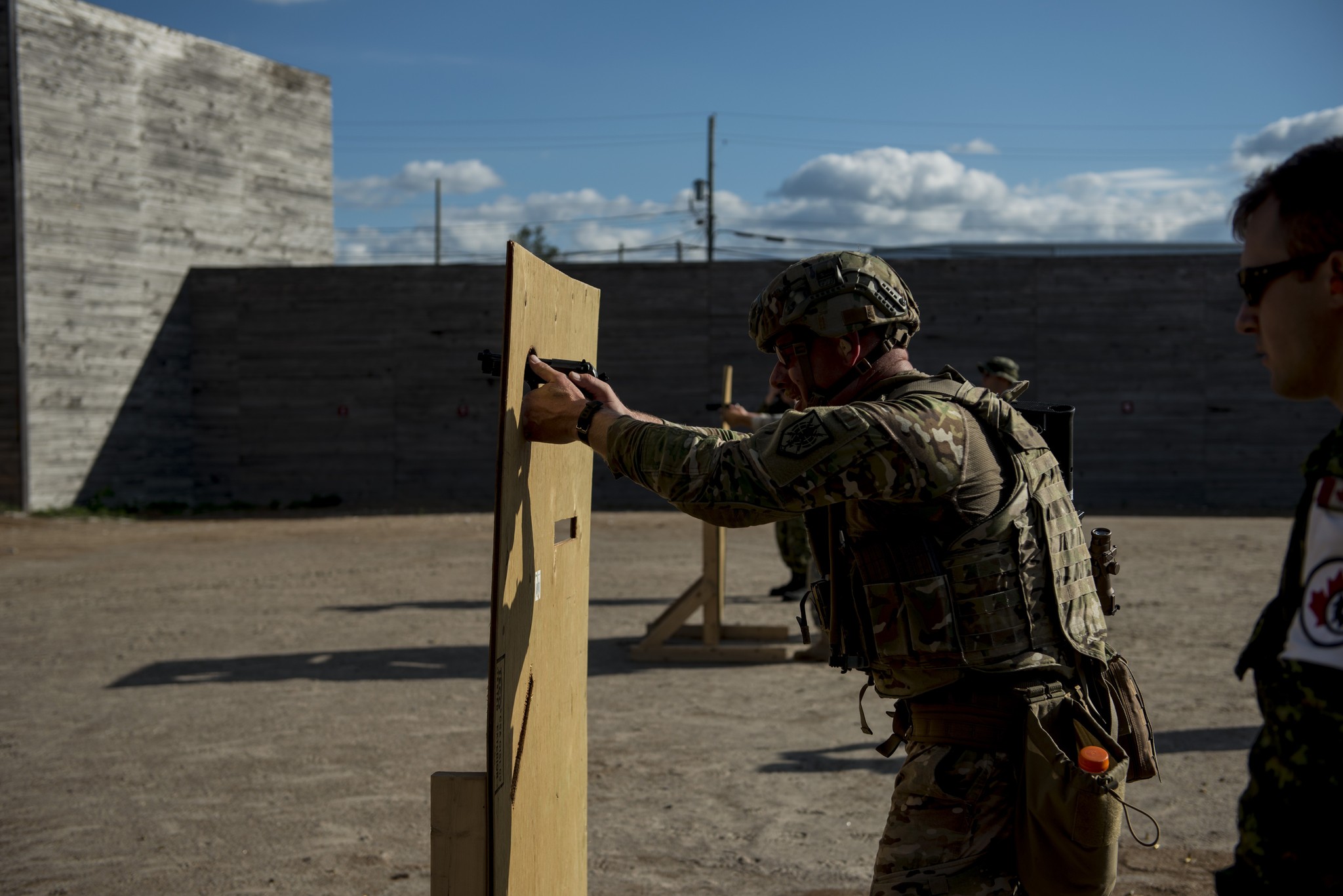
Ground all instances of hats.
[977,355,1019,383]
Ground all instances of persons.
[977,350,1019,400]
[723,382,812,603]
[522,255,1160,896]
[1207,133,1343,896]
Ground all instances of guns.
[706,400,740,413]
[481,349,608,400]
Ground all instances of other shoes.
[769,581,807,599]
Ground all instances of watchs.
[576,400,603,448]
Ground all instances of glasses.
[1236,246,1336,308]
[771,334,817,365]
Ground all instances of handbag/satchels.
[1024,694,1130,896]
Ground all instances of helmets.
[748,250,924,352]
